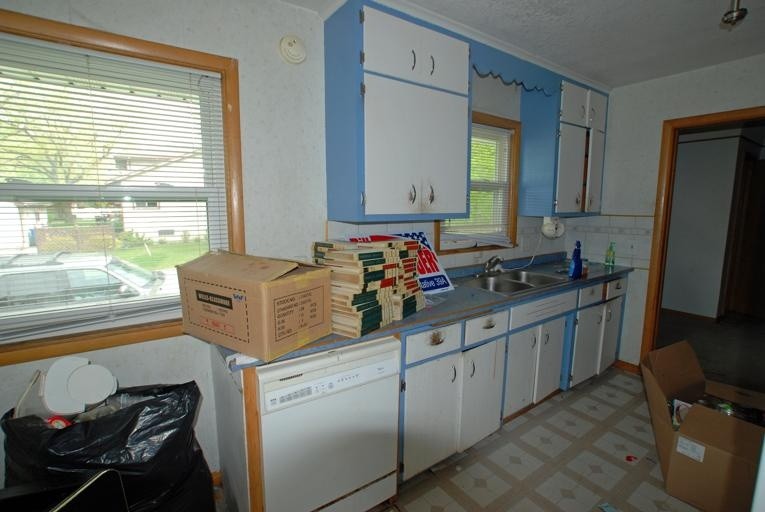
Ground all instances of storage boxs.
[177,248,331,363]
[640,340,765,512]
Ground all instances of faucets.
[473,254,504,278]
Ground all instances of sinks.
[498,269,564,287]
[464,276,534,295]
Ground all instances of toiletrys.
[604,241,616,266]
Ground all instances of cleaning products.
[568,240,582,280]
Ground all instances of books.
[311,233,427,340]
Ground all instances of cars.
[0,250,181,312]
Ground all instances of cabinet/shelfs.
[561,77,608,133]
[462,309,510,351]
[361,5,468,97]
[567,298,625,386]
[361,72,470,219]
[578,283,605,310]
[403,339,507,483]
[606,276,630,302]
[554,120,603,213]
[403,319,462,366]
[503,315,572,421]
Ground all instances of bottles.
[581,259,588,275]
